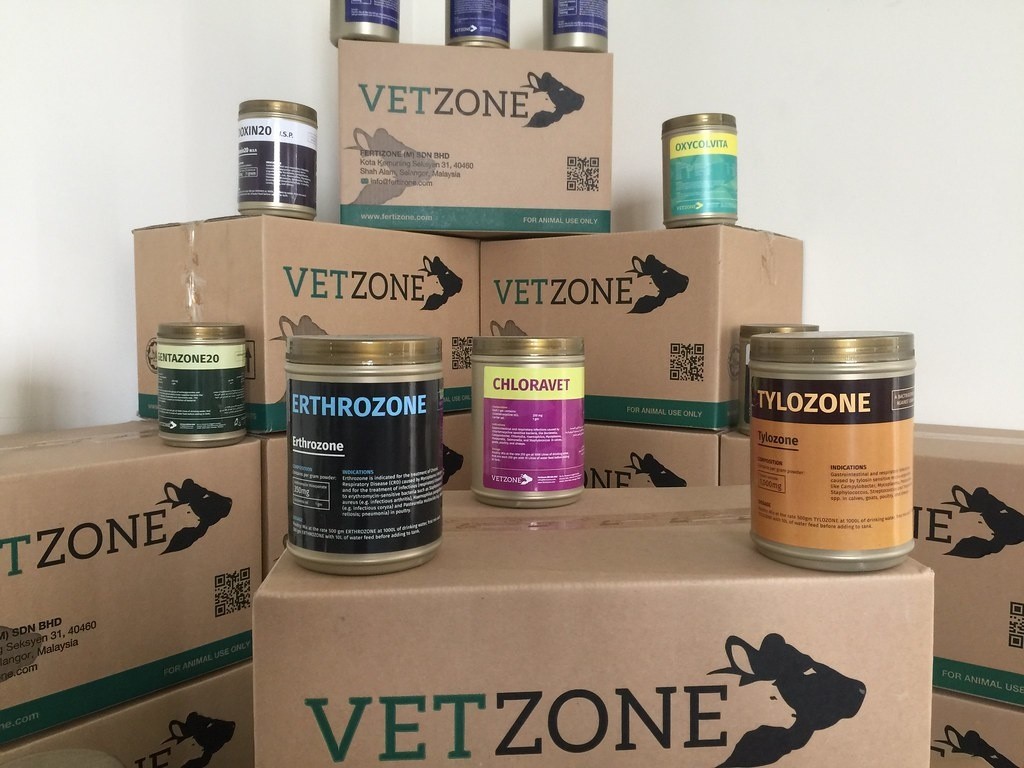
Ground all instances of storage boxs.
[478,222,804,432]
[0,419,262,749]
[930,687,1024,768]
[250,484,935,768]
[583,421,724,489]
[0,658,254,768]
[720,422,1024,707]
[246,410,472,581]
[131,213,480,435]
[337,37,613,240]
